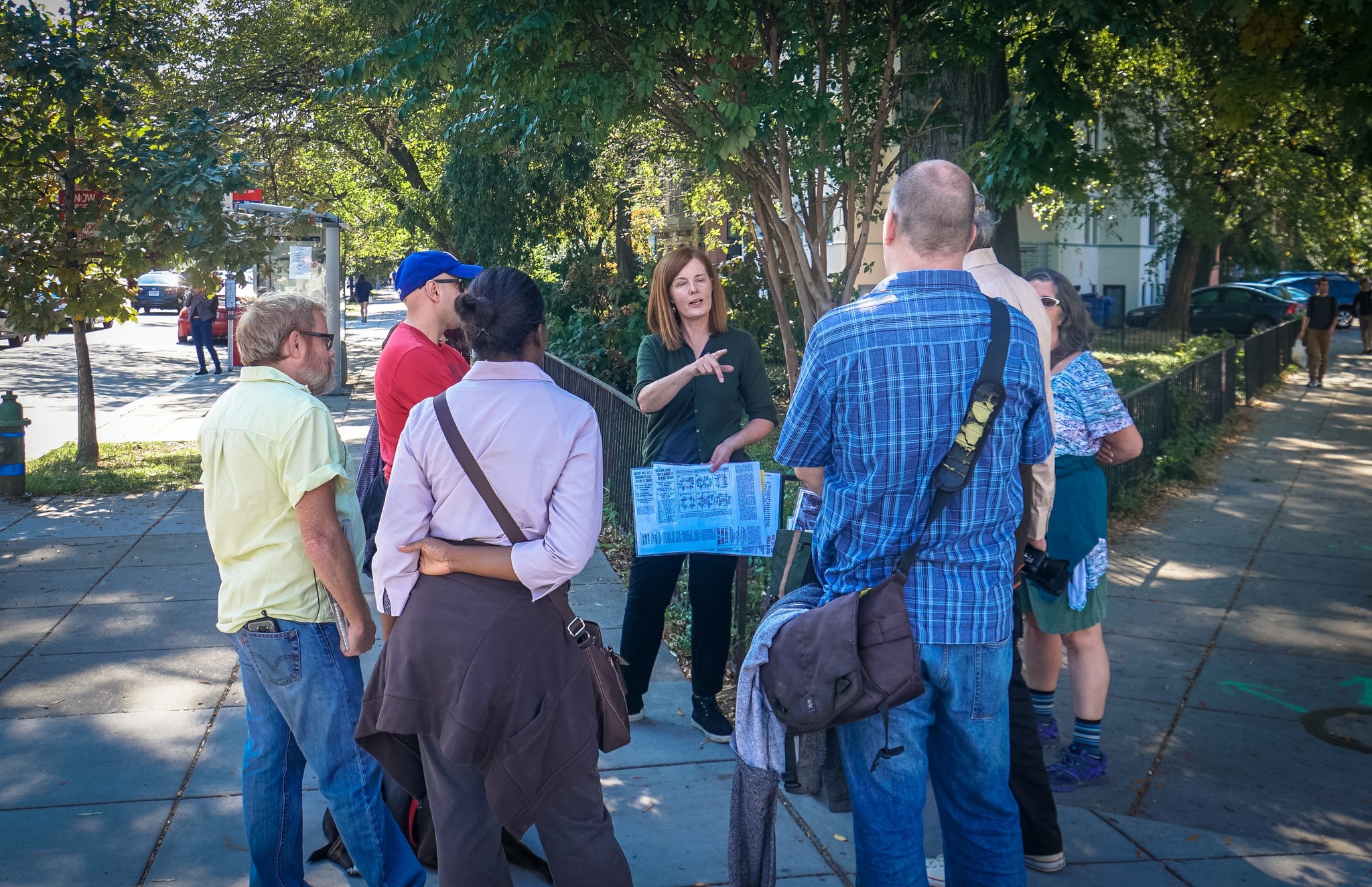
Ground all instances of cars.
[23,277,114,332]
[209,268,257,285]
[1257,271,1363,329]
[1123,283,1310,341]
[130,270,189,315]
[347,278,357,289]
[0,294,30,347]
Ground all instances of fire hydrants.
[0,390,33,502]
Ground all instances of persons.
[1350,278,1372,354]
[772,159,1055,887]
[375,250,483,485]
[185,271,223,375]
[392,270,396,281]
[196,292,426,887]
[354,273,373,323]
[1021,269,1143,792]
[618,248,779,742]
[1298,277,1340,387]
[925,182,1065,887]
[371,267,634,887]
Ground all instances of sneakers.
[692,696,733,743]
[1308,379,1318,387]
[214,367,222,374]
[1318,380,1323,385]
[195,368,208,375]
[626,695,644,721]
[1045,744,1106,793]
[1024,852,1066,872]
[1037,719,1061,749]
[924,855,945,887]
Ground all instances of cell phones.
[248,617,281,633]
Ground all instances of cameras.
[1021,545,1071,597]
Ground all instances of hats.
[394,251,484,301]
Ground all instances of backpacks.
[205,291,219,323]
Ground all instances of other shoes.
[361,320,364,323]
[1367,349,1372,355]
[1362,350,1367,355]
[364,317,367,323]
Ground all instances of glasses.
[1040,297,1060,308]
[299,331,334,351]
[419,278,465,292]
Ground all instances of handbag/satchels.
[758,570,925,735]
[579,621,631,753]
[1290,339,1308,368]
[368,294,373,303]
[322,768,438,876]
